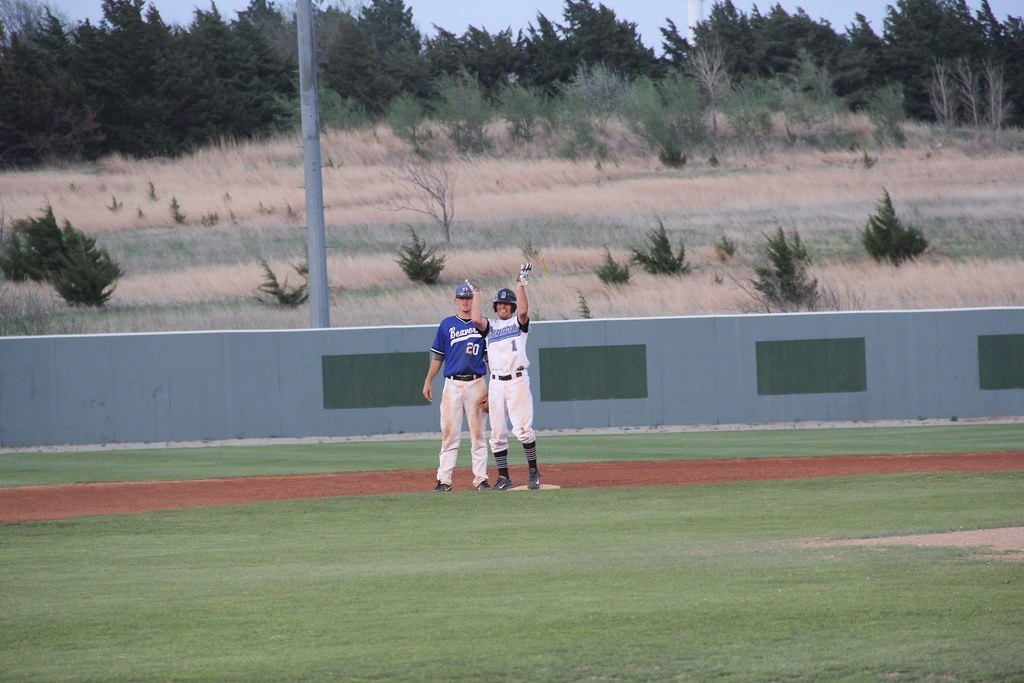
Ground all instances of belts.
[447,375,482,381]
[492,372,522,380]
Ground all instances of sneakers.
[527,468,540,489]
[491,476,513,490]
[432,480,453,492]
[476,480,491,490]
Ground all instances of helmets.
[492,288,517,313]
[456,284,473,298]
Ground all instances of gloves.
[465,278,479,292]
[516,262,533,286]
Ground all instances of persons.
[422,285,488,493]
[464,262,541,491]
